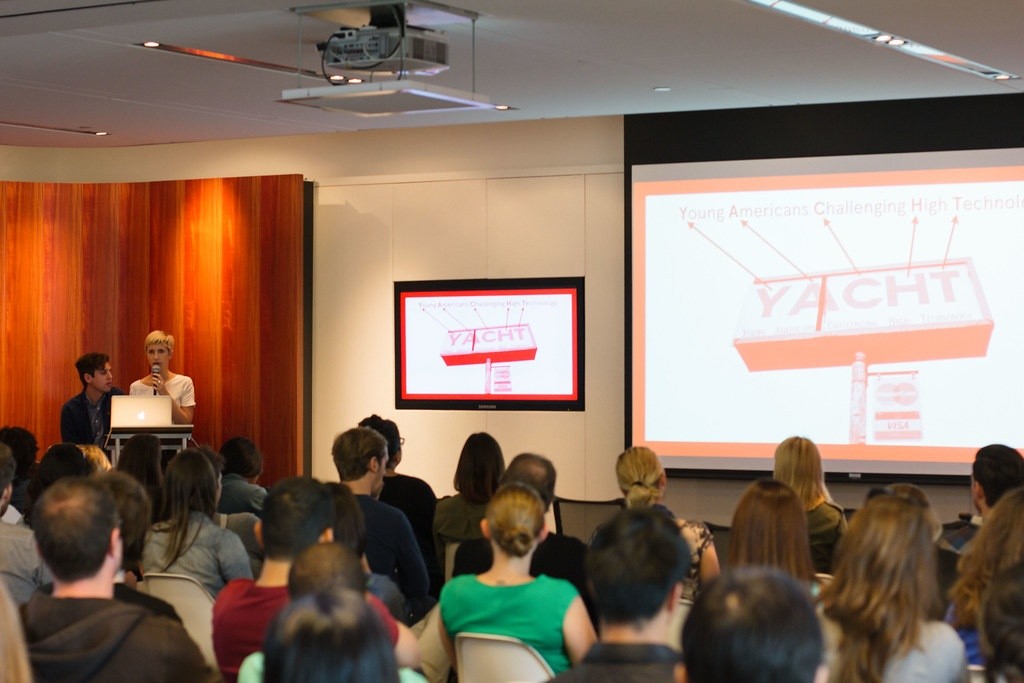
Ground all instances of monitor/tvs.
[395,276,585,412]
[110,395,172,427]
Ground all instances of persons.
[0,414,1024,683]
[60,330,127,463]
[128,330,195,475]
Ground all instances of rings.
[158,380,161,383]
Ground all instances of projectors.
[324,27,451,71]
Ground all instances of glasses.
[867,488,929,508]
[399,438,404,445]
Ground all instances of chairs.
[552,493,629,553]
[454,631,556,683]
[143,570,219,674]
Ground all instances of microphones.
[153,364,160,394]
